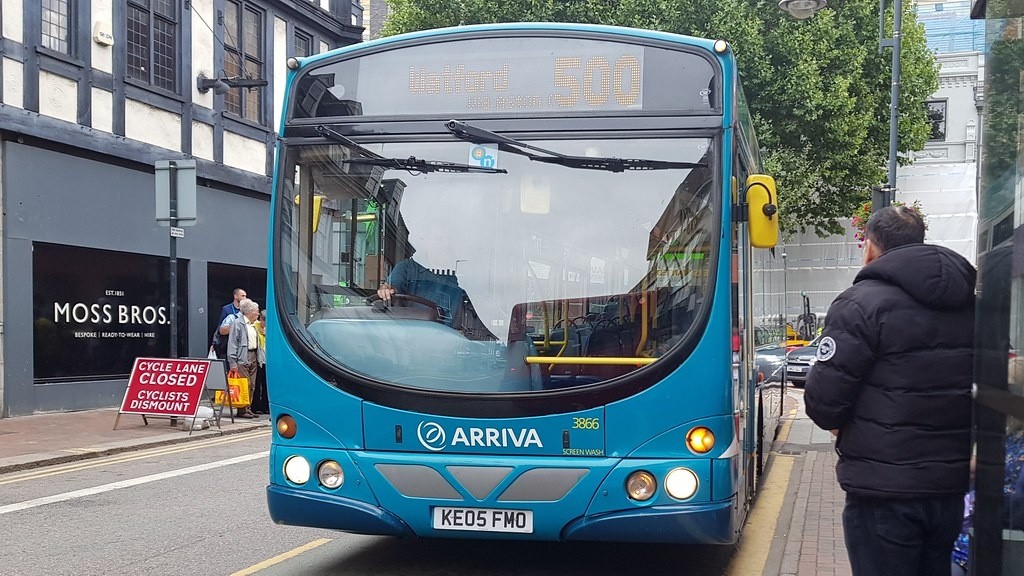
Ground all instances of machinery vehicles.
[785,291,817,351]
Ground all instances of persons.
[951,344,1024,576]
[377,229,484,330]
[213,288,271,419]
[804,205,984,576]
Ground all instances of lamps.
[214,69,237,95]
[935,3,943,11]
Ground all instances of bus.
[262,21,789,546]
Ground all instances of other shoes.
[247,411,259,418]
[237,411,253,418]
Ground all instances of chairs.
[547,296,642,378]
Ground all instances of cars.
[786,333,824,387]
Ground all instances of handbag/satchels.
[215,371,250,408]
[213,314,238,355]
[207,347,217,359]
